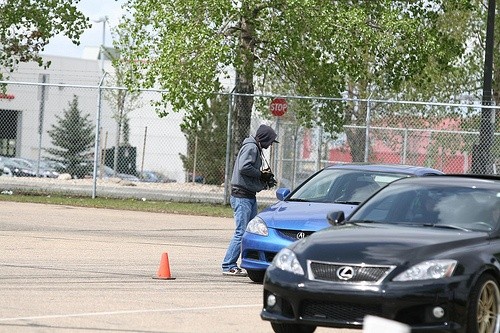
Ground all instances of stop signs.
[270,99,287,117]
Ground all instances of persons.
[222,124,279,277]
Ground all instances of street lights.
[92,15,108,198]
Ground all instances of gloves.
[267,178,274,188]
[260,167,274,180]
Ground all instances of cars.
[260,174,500,333]
[239,164,445,283]
[0,157,176,184]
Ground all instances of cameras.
[263,168,277,190]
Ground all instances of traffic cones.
[151,252,176,280]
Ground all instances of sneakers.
[222,268,247,276]
